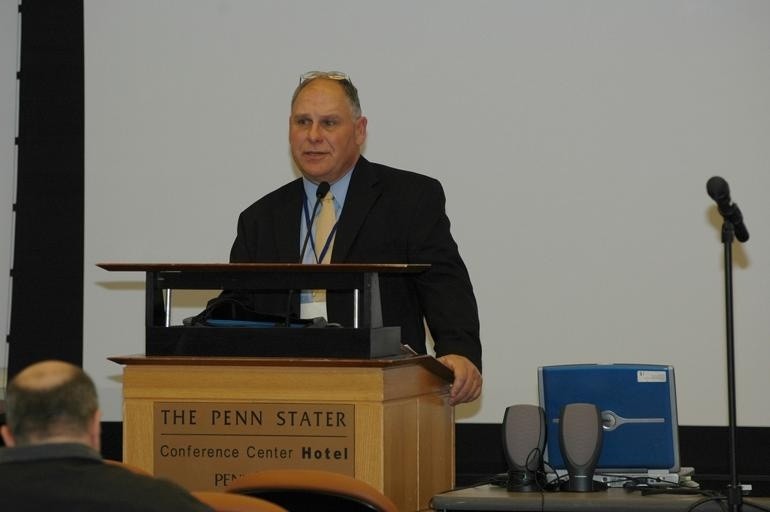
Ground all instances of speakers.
[558,403,608,492]
[500,405,555,492]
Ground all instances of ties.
[312,190,336,304]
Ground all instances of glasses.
[298,69,352,87]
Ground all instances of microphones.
[706,176,750,242]
[282,181,330,328]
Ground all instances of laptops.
[691,467,770,495]
[537,362,696,488]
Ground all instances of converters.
[491,473,524,486]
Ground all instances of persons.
[0,360,218,511]
[200,70,483,406]
